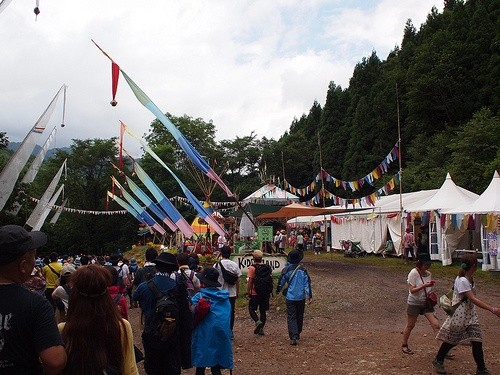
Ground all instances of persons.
[401,252,455,357]
[132,252,191,375]
[276,249,312,345]
[182,229,325,255]
[189,267,233,375]
[132,247,158,290]
[31,253,139,322]
[414,226,430,260]
[0,226,67,375]
[57,266,139,375]
[173,252,205,303]
[245,250,273,335]
[402,227,416,261]
[213,246,239,338]
[101,264,128,321]
[431,252,500,375]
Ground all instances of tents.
[223,185,346,232]
[285,171,500,271]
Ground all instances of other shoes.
[404,261,409,265]
[476,367,495,375]
[253,319,264,335]
[433,359,446,374]
[290,339,296,345]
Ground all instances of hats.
[61,272,72,277]
[287,249,304,264]
[251,250,263,259]
[0,225,47,265]
[418,253,431,266]
[222,246,231,257]
[405,228,412,232]
[129,259,137,265]
[155,252,177,266]
[195,267,223,287]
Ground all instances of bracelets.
[491,307,494,313]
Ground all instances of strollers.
[339,239,368,259]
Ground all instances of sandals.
[402,343,413,354]
[445,353,455,359]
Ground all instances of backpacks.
[404,234,413,248]
[175,270,196,301]
[251,264,274,298]
[141,264,157,281]
[143,279,184,354]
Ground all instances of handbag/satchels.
[191,291,211,329]
[133,344,144,363]
[215,259,240,285]
[425,289,438,309]
[282,281,289,296]
[439,294,455,315]
